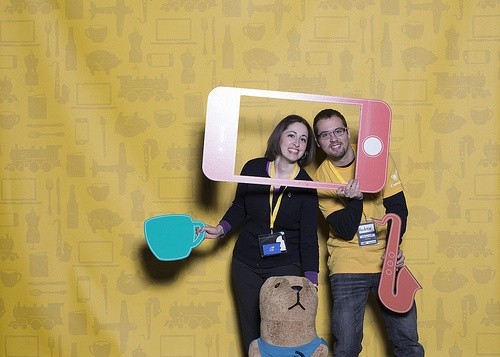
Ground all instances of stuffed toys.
[248,276,328,357]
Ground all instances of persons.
[312,108,425,357]
[196,115,320,357]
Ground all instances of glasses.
[317,127,349,140]
